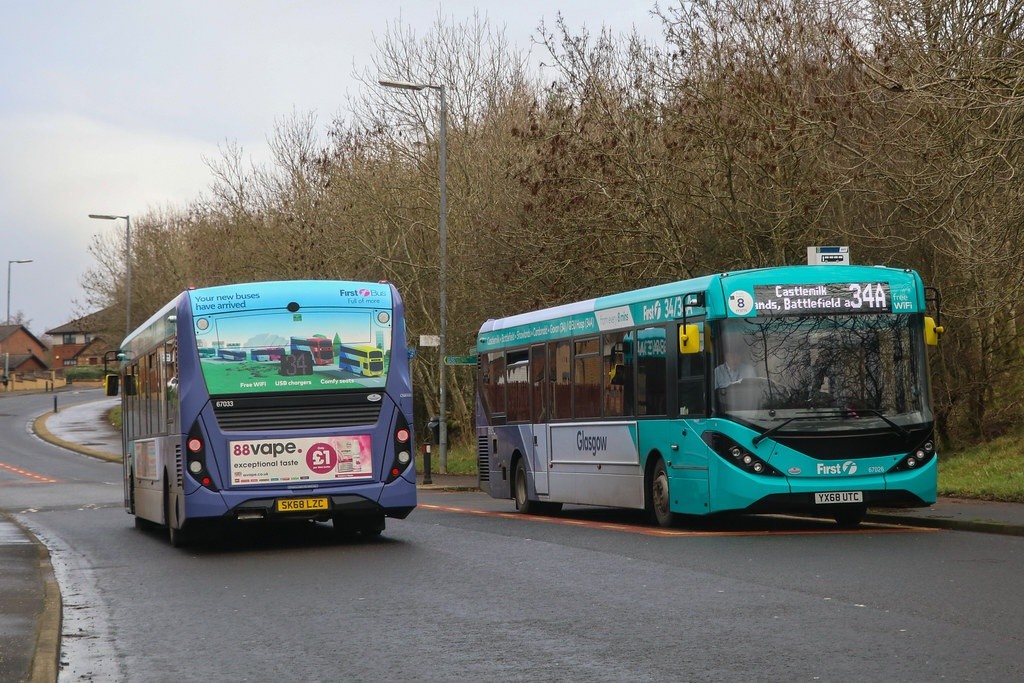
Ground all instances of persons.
[2,375,9,392]
[714,343,756,390]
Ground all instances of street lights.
[88,213,133,336]
[377,78,450,476]
[3,258,34,380]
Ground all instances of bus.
[102,277,419,544]
[473,262,947,532]
